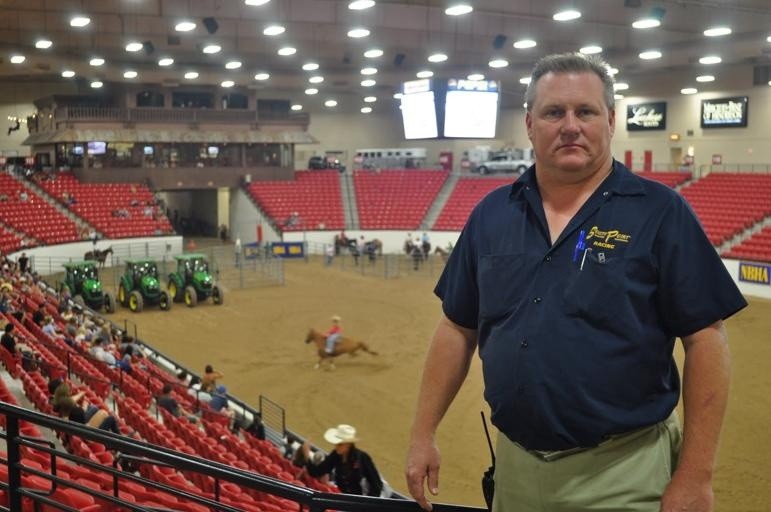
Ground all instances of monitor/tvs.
[627,102,666,129]
[88,141,107,154]
[72,147,84,156]
[208,146,219,154]
[701,96,748,128]
[144,146,154,154]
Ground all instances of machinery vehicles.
[60,260,115,313]
[118,253,224,312]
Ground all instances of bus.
[353,147,427,168]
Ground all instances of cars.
[308,156,346,172]
[476,151,534,175]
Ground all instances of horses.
[434,246,449,264]
[85,247,113,271]
[304,328,378,372]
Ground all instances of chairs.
[1,294,340,512]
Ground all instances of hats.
[324,424,359,444]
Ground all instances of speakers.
[650,8,666,20]
[168,35,180,45]
[394,54,404,64]
[624,1,641,8]
[753,65,771,85]
[203,17,218,33]
[492,33,507,49]
[143,40,154,55]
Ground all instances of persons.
[282,212,299,228]
[403,231,430,261]
[324,315,343,353]
[325,229,383,271]
[402,52,748,512]
[1,149,179,247]
[0,252,147,478]
[158,364,266,441]
[279,424,392,499]
